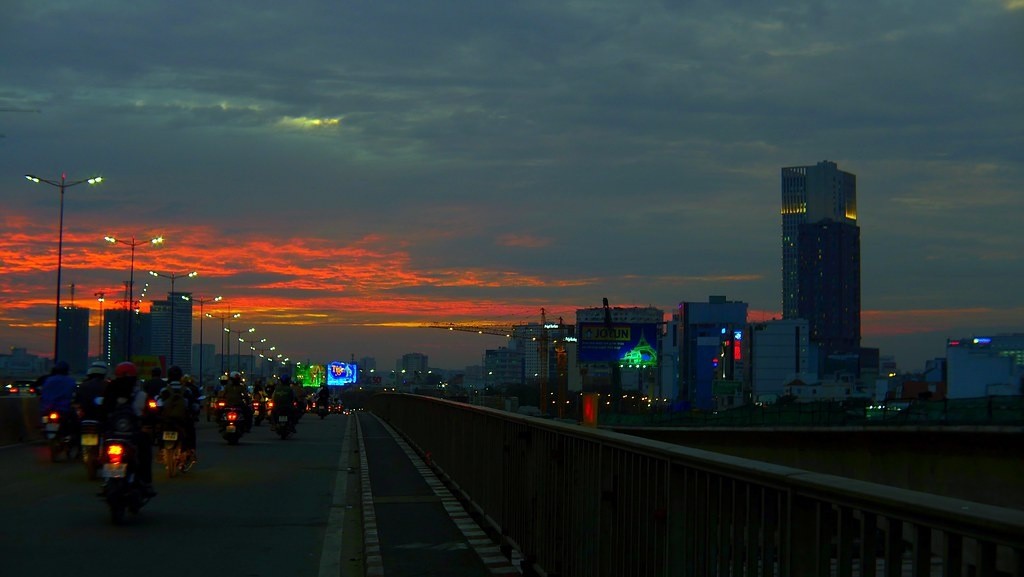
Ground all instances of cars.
[0,379,40,400]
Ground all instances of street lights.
[149,268,197,366]
[105,237,163,365]
[23,174,104,371]
[206,312,431,385]
[182,294,222,387]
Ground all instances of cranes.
[419,307,576,418]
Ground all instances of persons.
[31,359,351,534]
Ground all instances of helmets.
[115,361,139,378]
[56,361,70,372]
[86,361,108,376]
[152,365,328,388]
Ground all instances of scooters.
[38,399,363,524]
